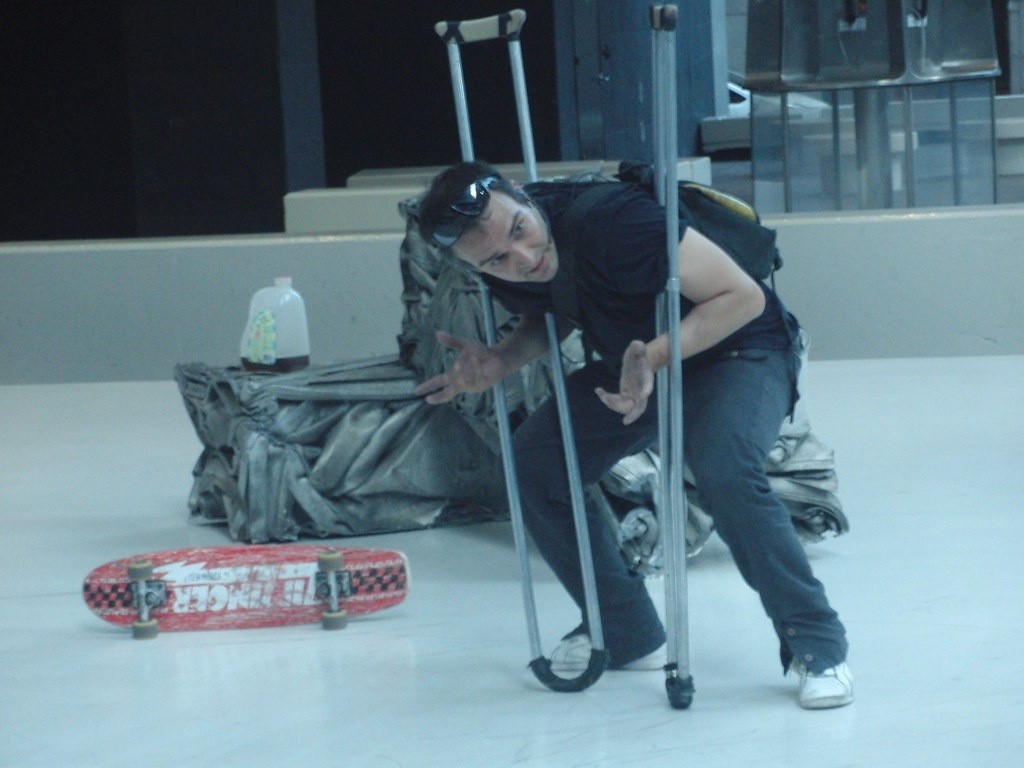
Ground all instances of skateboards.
[80,546,409,639]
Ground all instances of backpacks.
[522,157,784,329]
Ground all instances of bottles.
[238,277,310,377]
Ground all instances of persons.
[414,156,852,713]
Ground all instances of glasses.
[428,174,500,249]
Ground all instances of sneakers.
[546,632,667,671]
[791,658,855,710]
